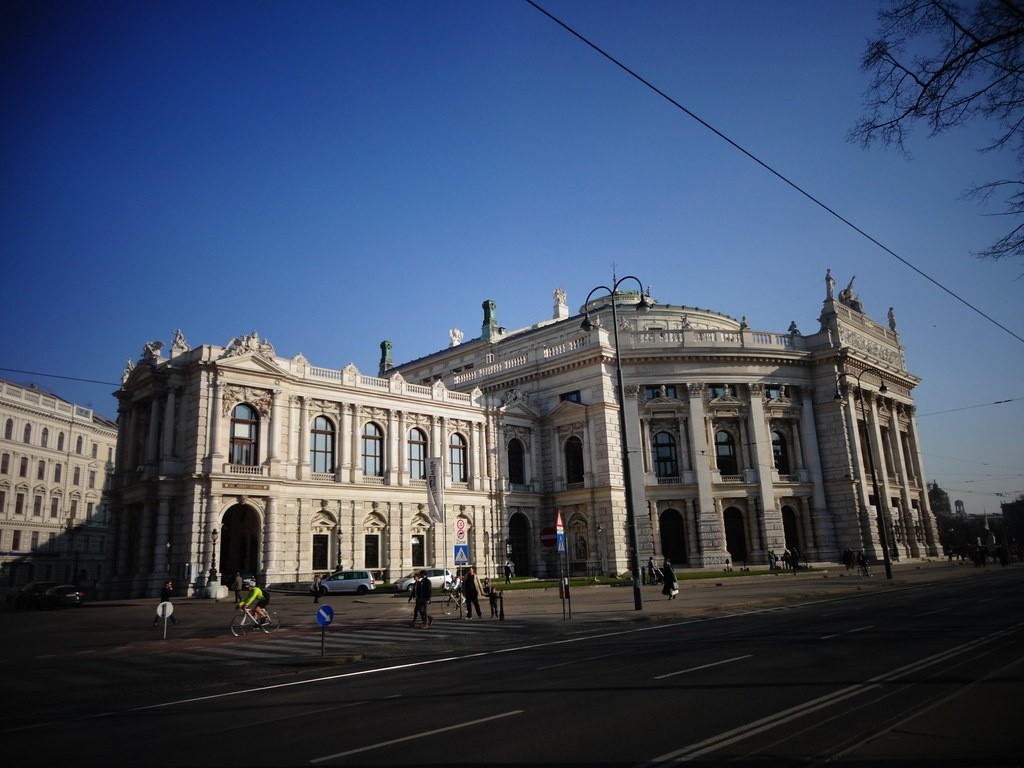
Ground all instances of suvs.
[13,582,56,610]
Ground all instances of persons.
[948,543,1024,568]
[844,283,866,316]
[232,572,243,604]
[408,570,434,629]
[768,547,800,575]
[448,567,500,620]
[825,269,835,299]
[662,557,677,600]
[153,580,183,627]
[648,556,656,586]
[237,582,270,630]
[843,547,869,573]
[888,307,896,332]
[313,574,323,603]
[787,321,800,334]
[504,563,512,584]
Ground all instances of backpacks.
[258,588,270,600]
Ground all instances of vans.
[310,570,376,596]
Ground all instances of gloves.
[242,605,247,610]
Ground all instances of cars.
[394,569,452,592]
[31,585,85,608]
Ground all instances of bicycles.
[230,604,281,637]
[441,586,467,615]
[857,560,874,579]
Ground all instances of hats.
[246,582,256,587]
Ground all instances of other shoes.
[408,623,414,627]
[464,617,472,620]
[174,620,180,625]
[153,624,159,627]
[428,616,433,625]
[420,625,427,628]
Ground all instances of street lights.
[580,263,653,608]
[834,369,893,580]
[207,529,218,581]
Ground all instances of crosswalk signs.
[454,545,468,564]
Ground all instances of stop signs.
[540,527,557,547]
